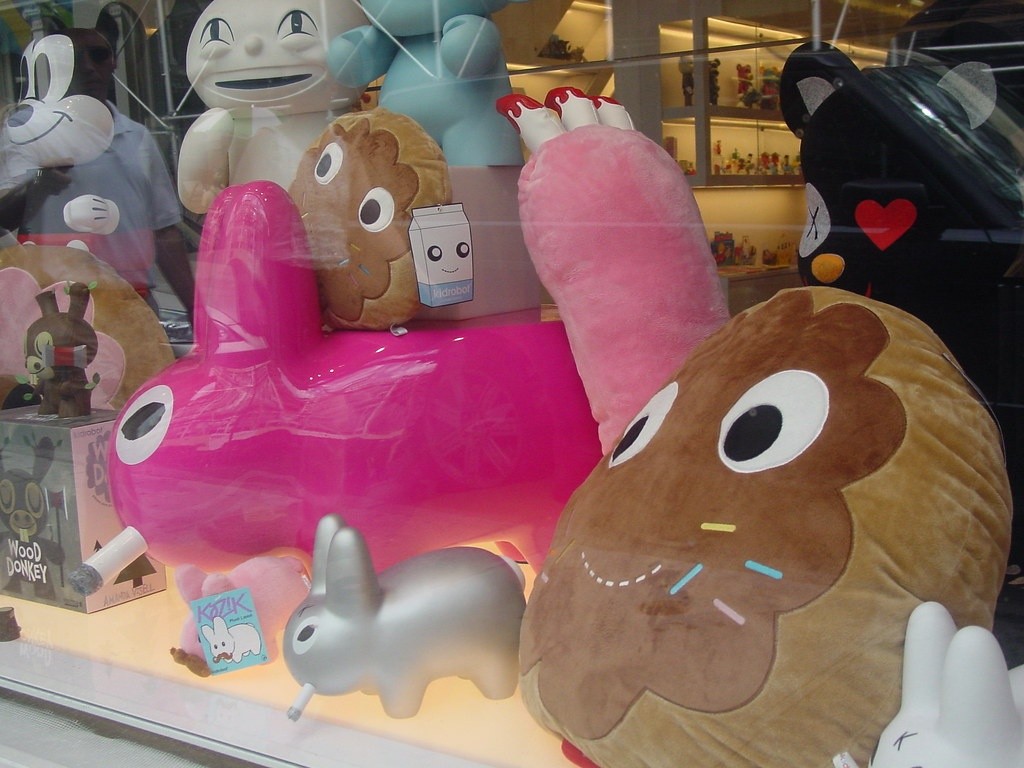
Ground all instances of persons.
[0,0,219,354]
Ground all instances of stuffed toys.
[287,105,454,336]
[779,41,1024,616]
[495,85,731,453]
[516,286,1024,768]
[0,245,175,412]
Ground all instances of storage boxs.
[0,406,167,613]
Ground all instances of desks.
[717,267,791,316]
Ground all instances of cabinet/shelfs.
[662,106,807,186]
